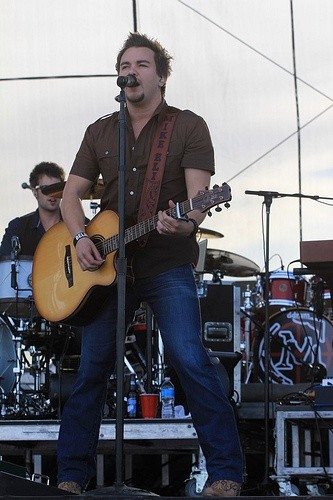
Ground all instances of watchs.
[72,232,89,248]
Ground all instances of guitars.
[31,182,231,322]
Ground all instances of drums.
[0,254,40,319]
[257,270,307,317]
[251,306,333,384]
[1,313,20,397]
[18,315,75,344]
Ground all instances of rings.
[164,228,167,233]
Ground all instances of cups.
[140,394,159,419]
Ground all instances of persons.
[0,161,91,261]
[57,31,247,500]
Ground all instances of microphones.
[116,75,137,87]
[12,236,21,254]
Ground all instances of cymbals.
[194,227,224,239]
[206,249,260,277]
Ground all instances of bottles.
[160,377,175,418]
[126,374,138,419]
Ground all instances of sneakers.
[203,480,241,496]
[58,481,83,494]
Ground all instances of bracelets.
[189,218,199,238]
